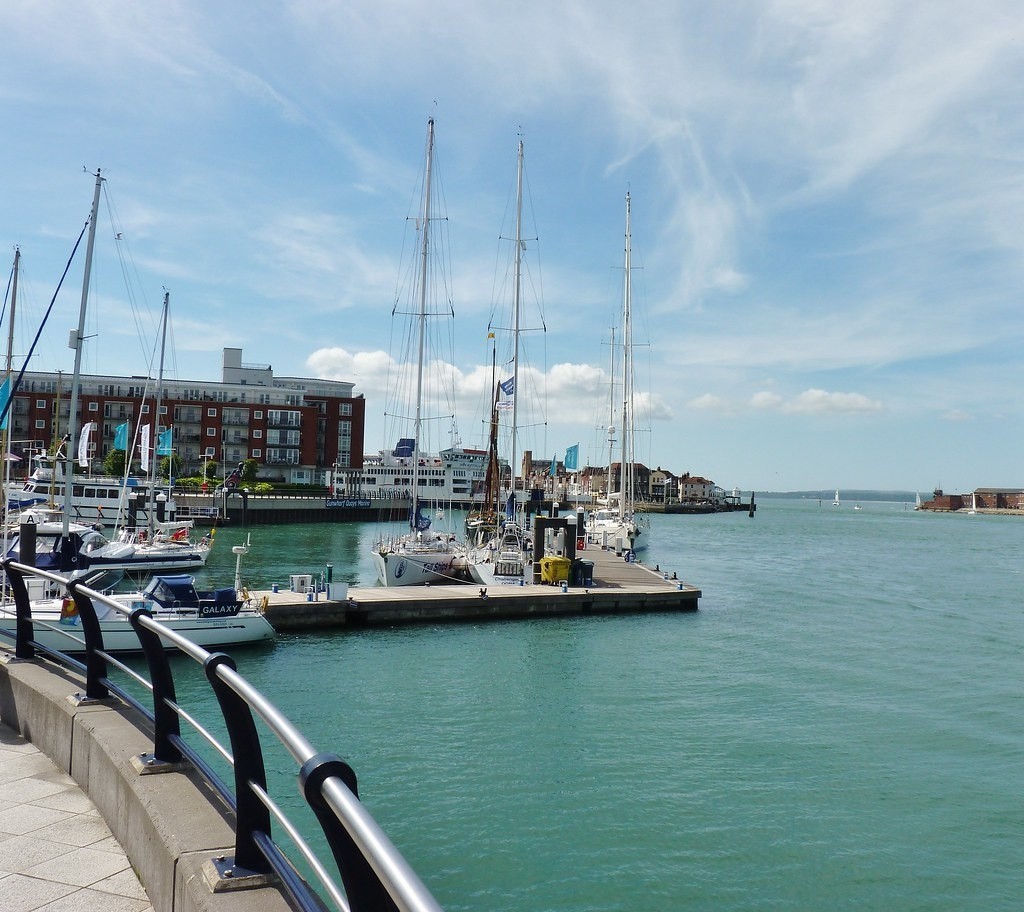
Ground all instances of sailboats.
[371,111,652,585]
[2,167,228,571]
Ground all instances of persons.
[378,487,413,499]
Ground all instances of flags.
[488,332,495,338]
[500,377,513,394]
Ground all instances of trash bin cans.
[271,583,279,593]
[306,592,313,602]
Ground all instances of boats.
[0,574,278,650]
